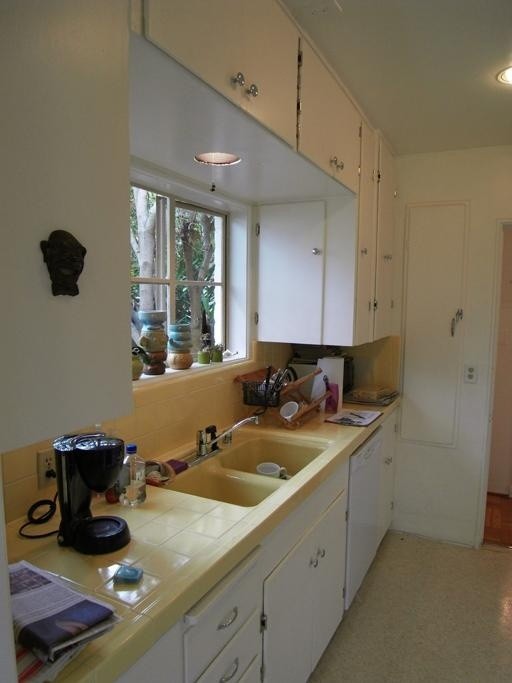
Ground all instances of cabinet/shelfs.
[185,545,262,683]
[404,197,468,449]
[118,618,184,682]
[345,408,398,612]
[263,489,345,682]
[142,1,360,193]
[255,124,395,347]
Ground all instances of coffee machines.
[52,431,131,555]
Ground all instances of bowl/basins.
[143,352,167,375]
[138,309,168,325]
[166,323,192,370]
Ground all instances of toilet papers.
[309,355,344,413]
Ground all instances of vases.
[131,310,223,381]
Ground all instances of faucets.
[198,415,260,456]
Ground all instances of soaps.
[115,564,143,582]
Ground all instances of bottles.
[131,354,143,381]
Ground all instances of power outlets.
[37,448,58,490]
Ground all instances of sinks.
[159,464,282,511]
[213,430,330,484]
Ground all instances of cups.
[211,350,222,362]
[198,351,210,364]
[256,462,287,479]
[280,401,300,423]
